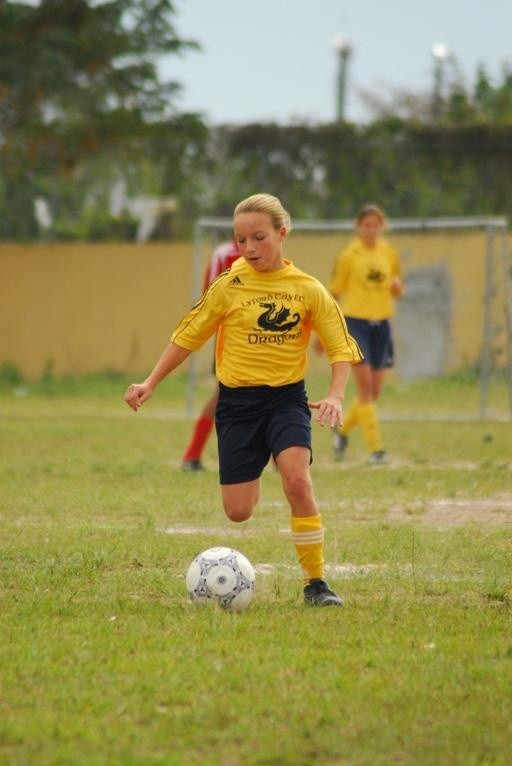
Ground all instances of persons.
[179,229,242,474]
[124,194,364,608]
[312,205,405,471]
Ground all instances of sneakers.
[304,578,343,606]
[334,426,348,458]
[182,460,206,471]
[370,452,387,463]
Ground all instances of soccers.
[187,547,255,612]
